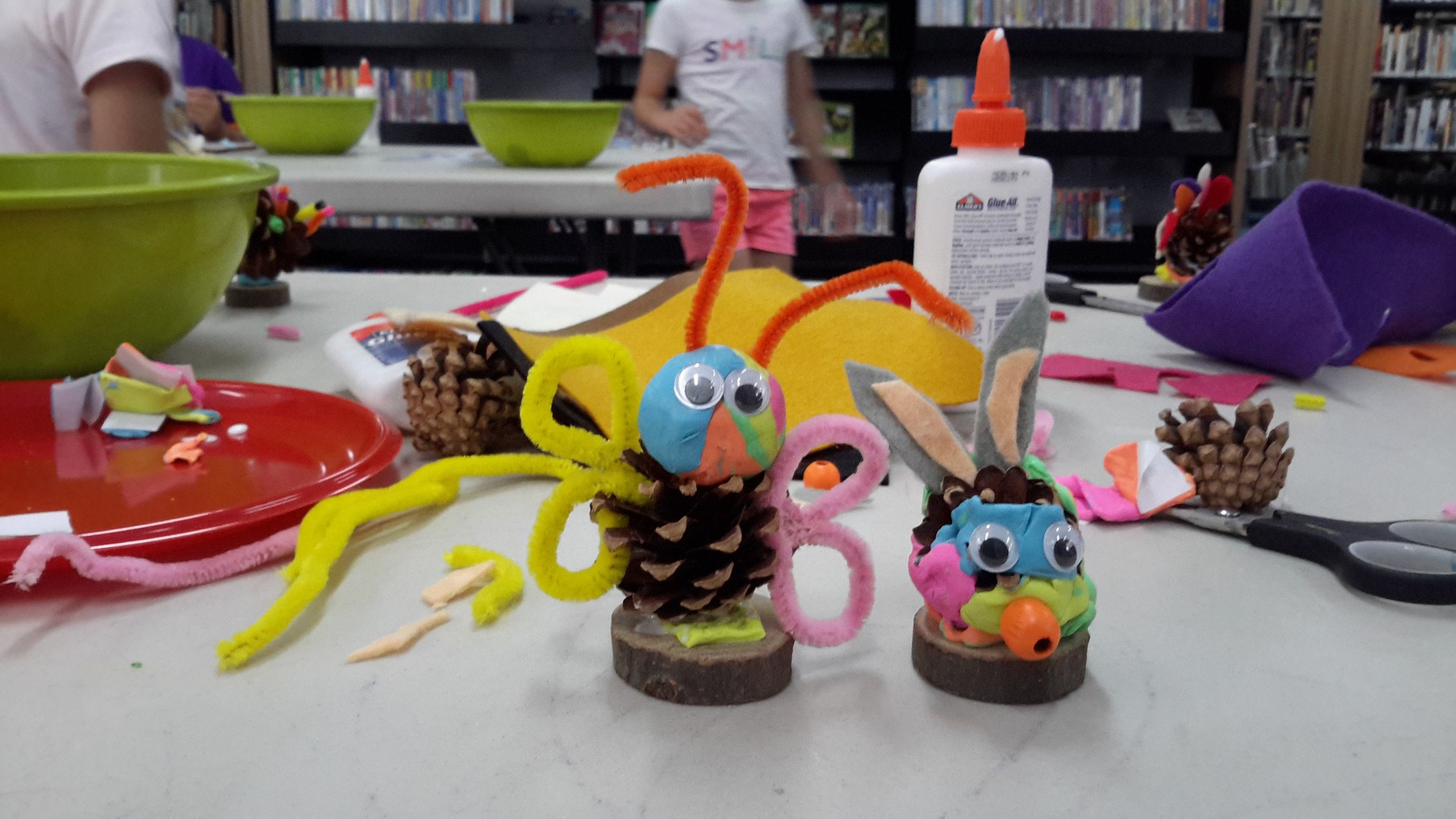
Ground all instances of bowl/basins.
[461,101,622,166]
[1,154,277,382]
[226,95,380,156]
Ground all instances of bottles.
[322,314,481,429]
[351,59,380,154]
[908,29,1052,410]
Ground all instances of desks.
[0,266,1456,819]
[222,143,715,268]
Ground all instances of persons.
[632,0,858,275]
[0,0,199,155]
[177,33,246,141]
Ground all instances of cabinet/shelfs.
[238,0,1456,273]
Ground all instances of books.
[907,0,1224,240]
[275,0,513,122]
[178,0,213,44]
[1365,23,1456,149]
[1247,0,1322,194]
[787,5,892,236]
[319,217,475,229]
[550,219,679,235]
[597,2,644,55]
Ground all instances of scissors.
[1163,502,1456,607]
[1041,271,1156,317]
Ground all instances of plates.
[0,378,403,573]
